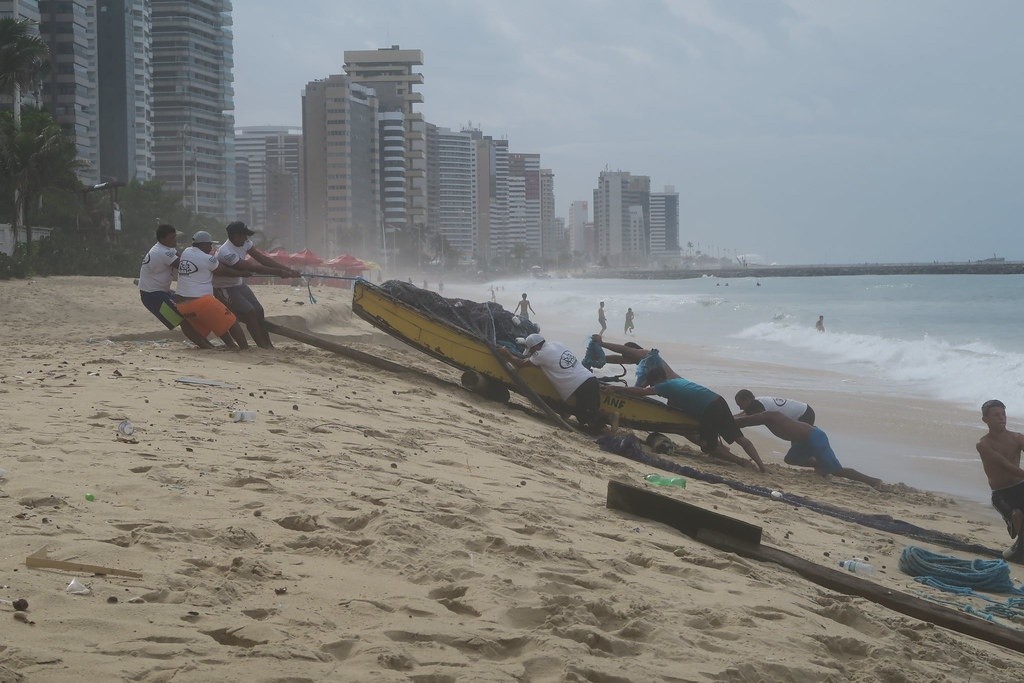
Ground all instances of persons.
[729,399,883,487]
[514,293,536,319]
[139,221,217,350]
[596,301,609,338]
[816,313,825,329]
[176,231,248,350]
[976,400,1023,557]
[623,307,634,334]
[731,390,816,444]
[212,221,300,348]
[587,334,765,474]
[496,333,621,434]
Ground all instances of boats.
[351,278,706,435]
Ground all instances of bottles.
[840,560,874,575]
[644,474,686,487]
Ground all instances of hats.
[522,333,545,355]
[226,221,255,236]
[192,231,219,244]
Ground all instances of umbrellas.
[247,245,370,276]
